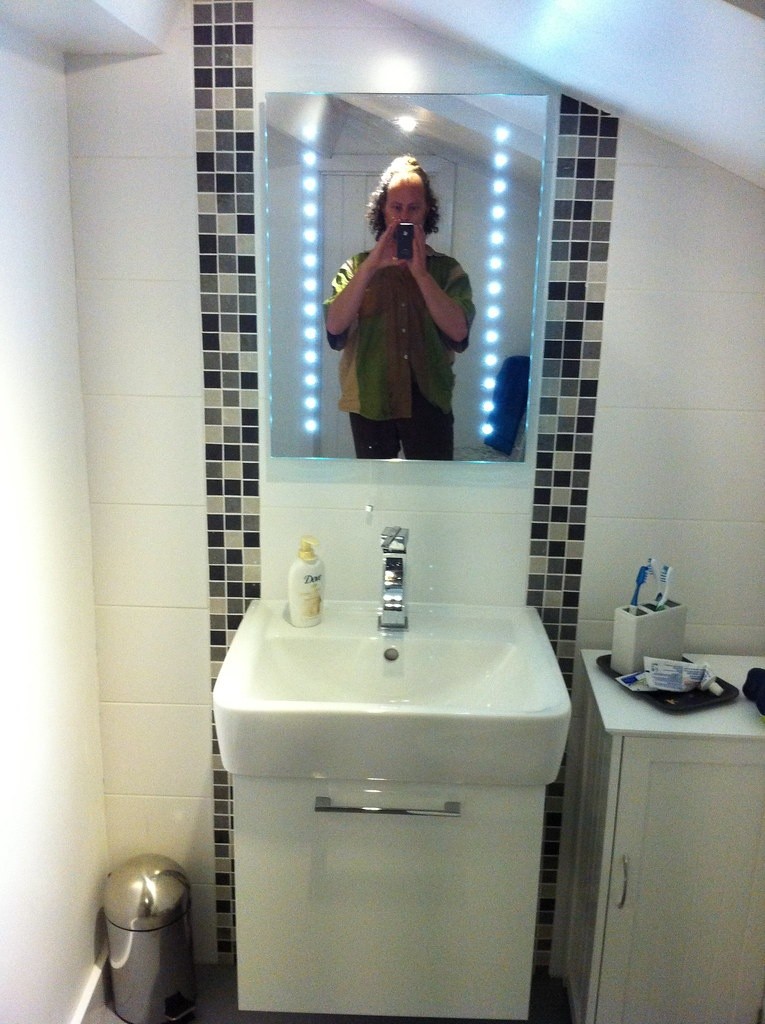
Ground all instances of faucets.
[379,526,412,633]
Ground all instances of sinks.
[213,600,572,787]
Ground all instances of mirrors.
[263,90,550,463]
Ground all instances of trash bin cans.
[101,853,198,1024]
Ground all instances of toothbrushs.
[656,564,675,611]
[647,557,663,600]
[628,566,650,615]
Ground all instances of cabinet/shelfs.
[563,648,765,1024]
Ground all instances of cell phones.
[396,223,414,260]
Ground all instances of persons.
[320,151,480,460]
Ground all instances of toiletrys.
[644,657,725,696]
[616,669,659,693]
[289,535,325,625]
